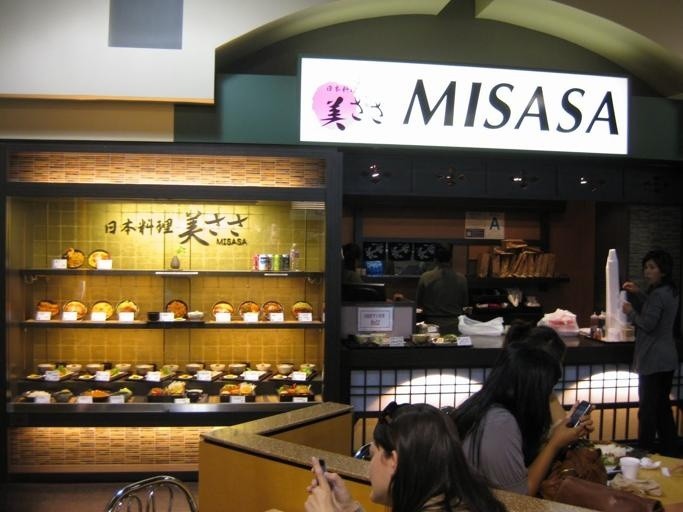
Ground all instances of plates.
[563,464,622,474]
[115,298,139,319]
[166,299,188,318]
[211,301,234,320]
[62,300,87,320]
[124,372,178,384]
[71,373,131,383]
[26,374,72,382]
[37,300,60,318]
[87,250,111,269]
[64,250,85,269]
[220,371,272,383]
[91,299,113,320]
[458,337,472,346]
[262,300,284,320]
[172,371,224,382]
[576,445,634,452]
[238,301,261,321]
[291,301,313,320]
[432,338,458,346]
[371,341,406,347]
[268,370,318,383]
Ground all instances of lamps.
[367,159,376,169]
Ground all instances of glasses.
[377,401,397,425]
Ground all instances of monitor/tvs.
[343,282,385,302]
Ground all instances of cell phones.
[566,400,591,428]
[319,459,325,472]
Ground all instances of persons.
[448,342,585,498]
[622,251,681,457]
[304,401,508,512]
[417,241,469,337]
[341,244,363,283]
[502,324,596,439]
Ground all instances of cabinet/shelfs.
[466,239,571,321]
[1,182,327,415]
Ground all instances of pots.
[355,335,371,344]
[370,333,386,343]
[410,334,429,344]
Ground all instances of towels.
[609,473,666,499]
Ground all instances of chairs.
[356,442,372,457]
[109,476,196,511]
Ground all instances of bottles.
[268,254,274,270]
[282,255,290,271]
[259,254,269,270]
[599,311,606,337]
[290,243,300,271]
[274,255,282,271]
[591,312,599,336]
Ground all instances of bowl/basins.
[210,364,225,372]
[220,391,256,402]
[80,391,112,402]
[163,365,179,375]
[228,364,246,374]
[300,364,316,372]
[51,392,73,402]
[87,364,104,375]
[186,364,203,375]
[280,392,314,402]
[37,364,54,375]
[136,365,154,375]
[147,389,203,402]
[66,364,81,376]
[22,390,52,402]
[116,364,131,375]
[147,312,159,321]
[188,311,203,320]
[256,364,271,373]
[276,364,293,374]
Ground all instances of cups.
[623,326,634,341]
[620,290,626,305]
[620,457,640,481]
[101,363,111,371]
[150,364,156,371]
[198,362,205,369]
[596,328,602,340]
[55,361,63,369]
[606,249,620,341]
[242,362,250,369]
